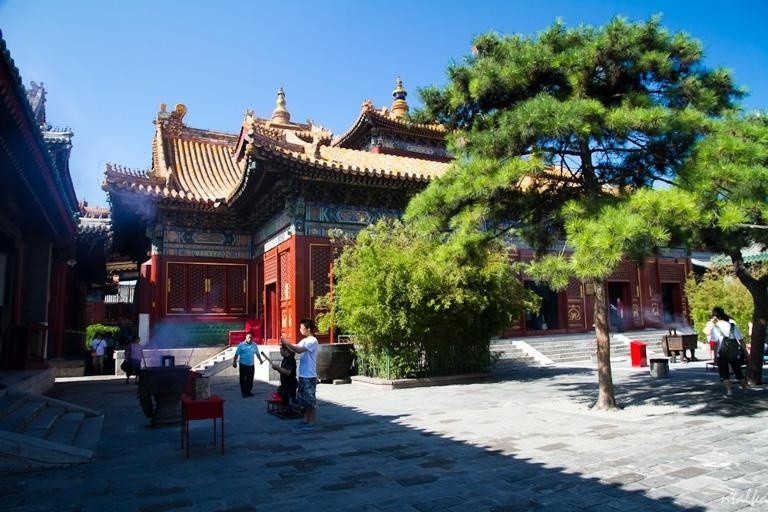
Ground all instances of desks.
[181,394,225,459]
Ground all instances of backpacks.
[713,323,742,361]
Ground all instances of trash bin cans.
[650,359,669,380]
[160,355,175,367]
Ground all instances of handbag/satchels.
[92,352,96,357]
[121,360,131,372]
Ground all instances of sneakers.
[723,394,734,399]
[295,420,317,431]
[243,392,254,398]
[740,382,750,392]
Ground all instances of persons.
[233,332,264,398]
[281,320,319,434]
[271,342,298,404]
[697,306,751,401]
[87,330,118,374]
[614,298,627,335]
[124,335,147,385]
